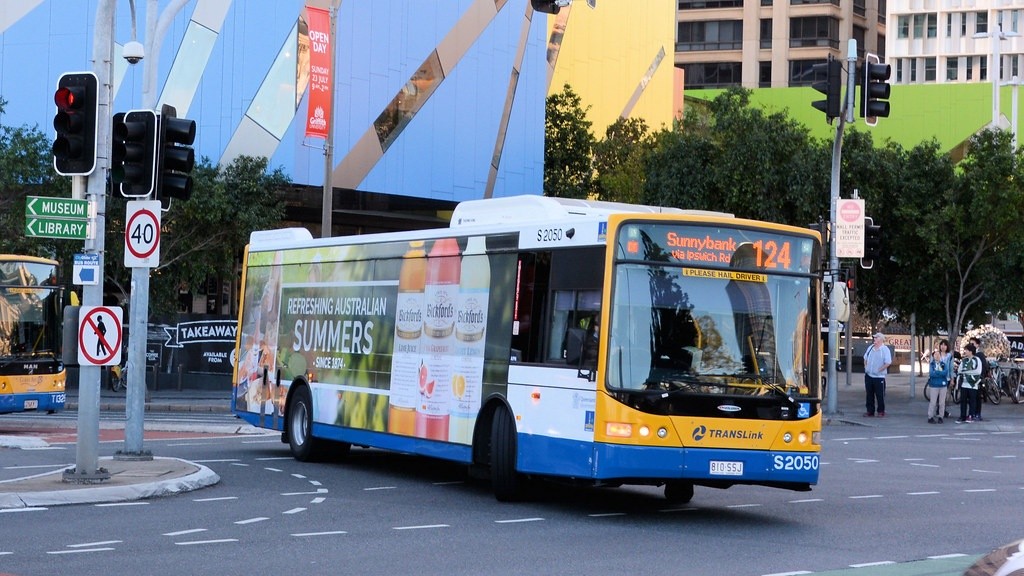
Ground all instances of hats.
[872,332,885,342]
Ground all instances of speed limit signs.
[126,210,160,258]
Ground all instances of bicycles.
[924,353,1024,405]
[110,360,127,392]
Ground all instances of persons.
[577,311,601,339]
[863,332,893,418]
[925,339,988,423]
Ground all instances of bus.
[230,194,850,502]
[0,254,80,414]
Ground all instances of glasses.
[968,342,975,346]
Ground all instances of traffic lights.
[811,53,840,118]
[52,72,99,175]
[114,109,157,197]
[155,103,196,212]
[864,53,890,126]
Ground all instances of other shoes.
[954,413,983,423]
[863,412,874,417]
[928,418,936,424]
[944,411,951,418]
[935,412,940,417]
[938,417,944,424]
[877,411,886,417]
